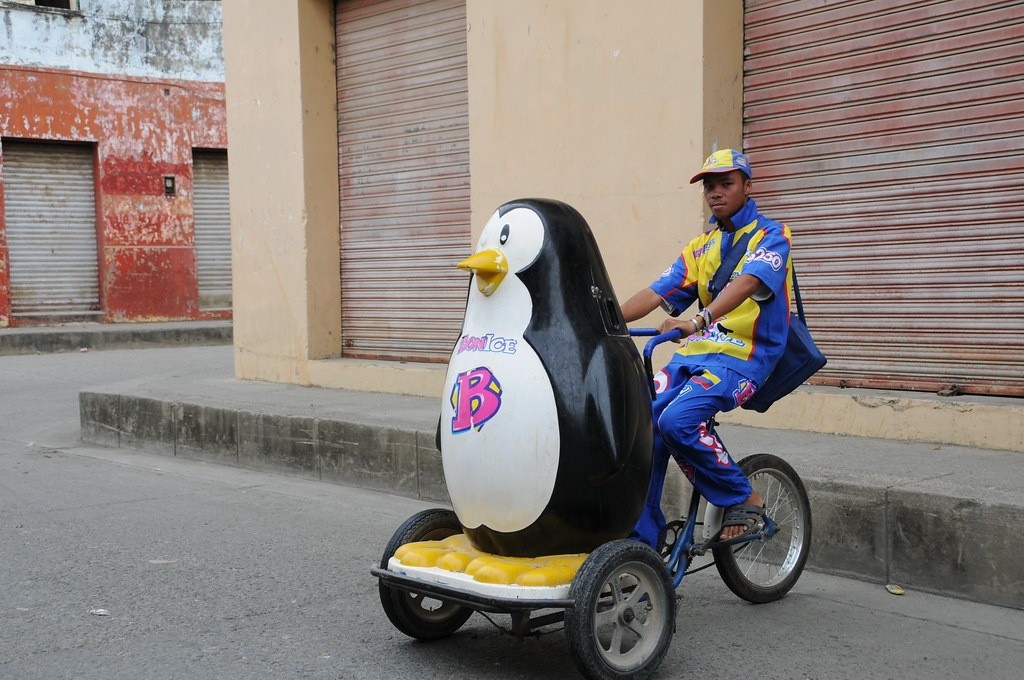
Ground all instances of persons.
[617,148,793,551]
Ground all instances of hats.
[689,149,751,184]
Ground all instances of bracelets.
[690,318,699,332]
[696,308,713,327]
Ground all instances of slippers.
[718,503,767,544]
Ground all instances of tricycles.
[369,322,815,680]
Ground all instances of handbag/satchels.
[709,219,826,413]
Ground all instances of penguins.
[392,197,655,585]
